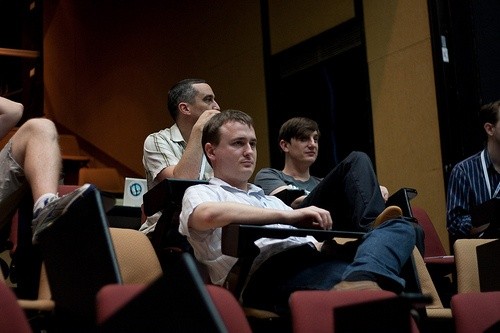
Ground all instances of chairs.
[0,129,500,333]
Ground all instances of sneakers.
[30,182,91,246]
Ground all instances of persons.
[0,97,93,247]
[447,109,500,256]
[178,109,416,296]
[254,117,403,230]
[138,79,223,239]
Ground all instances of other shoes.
[373,205,403,227]
[330,279,383,293]
